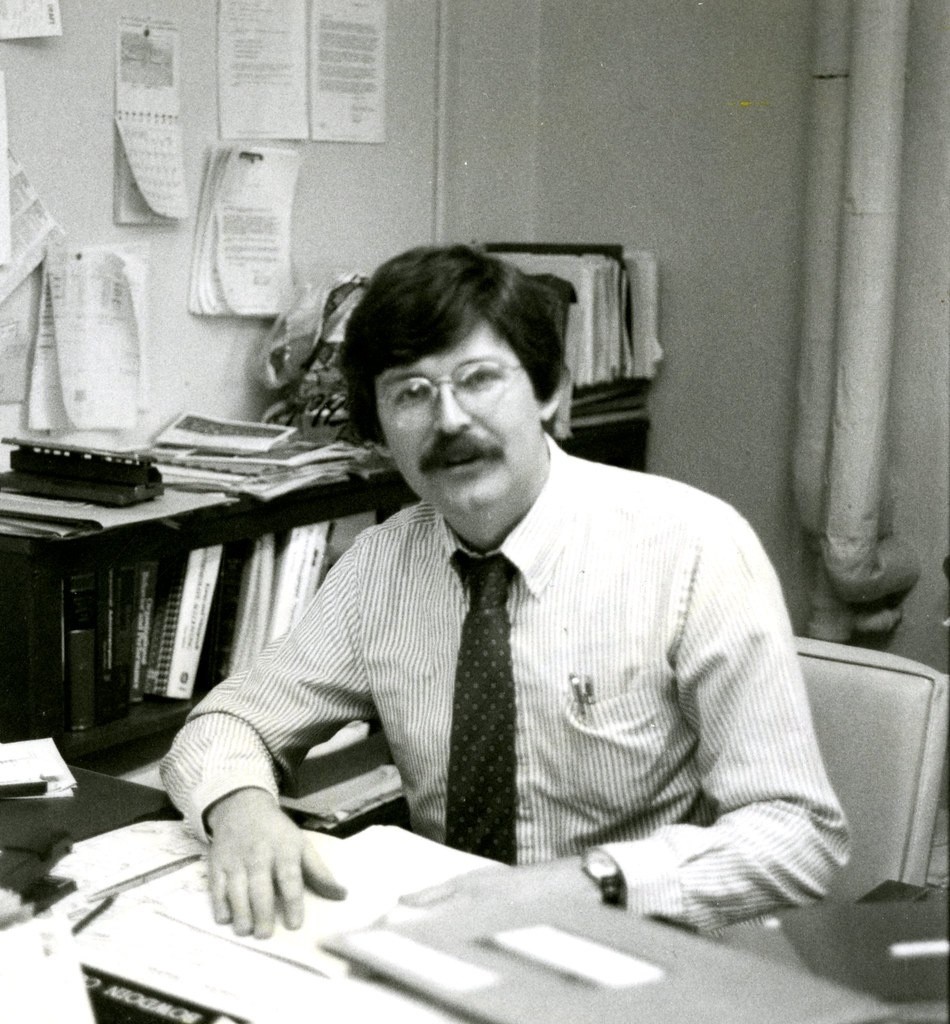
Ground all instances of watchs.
[581,846,625,911]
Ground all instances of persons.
[161,246,851,939]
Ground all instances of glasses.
[371,357,525,428]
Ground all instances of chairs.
[754,634,948,926]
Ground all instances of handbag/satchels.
[260,270,372,446]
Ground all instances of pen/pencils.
[569,672,596,719]
[71,890,120,934]
[89,854,201,901]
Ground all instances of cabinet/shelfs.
[0,389,653,768]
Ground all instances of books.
[57,521,333,730]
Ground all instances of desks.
[0,762,948,1022]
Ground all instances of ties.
[443,548,518,870]
